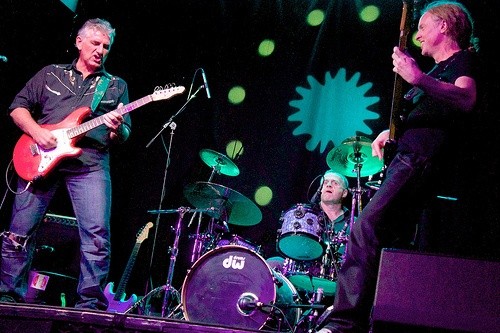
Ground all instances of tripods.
[121,205,213,316]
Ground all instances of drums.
[228,233,265,258]
[278,204,327,262]
[265,256,286,277]
[281,245,344,296]
[180,244,302,333]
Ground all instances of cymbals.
[326,135,387,178]
[364,181,383,190]
[199,149,241,177]
[147,207,221,214]
[183,181,263,227]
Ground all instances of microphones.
[239,297,263,310]
[311,181,325,202]
[201,69,211,98]
[0,55,8,62]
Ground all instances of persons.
[0,13,133,312]
[300,1,487,333]
[288,169,362,311]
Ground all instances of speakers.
[364,247,499,333]
[30,214,81,279]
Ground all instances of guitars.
[378,0,419,244]
[12,82,186,185]
[101,221,154,315]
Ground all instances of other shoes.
[1,295,17,304]
